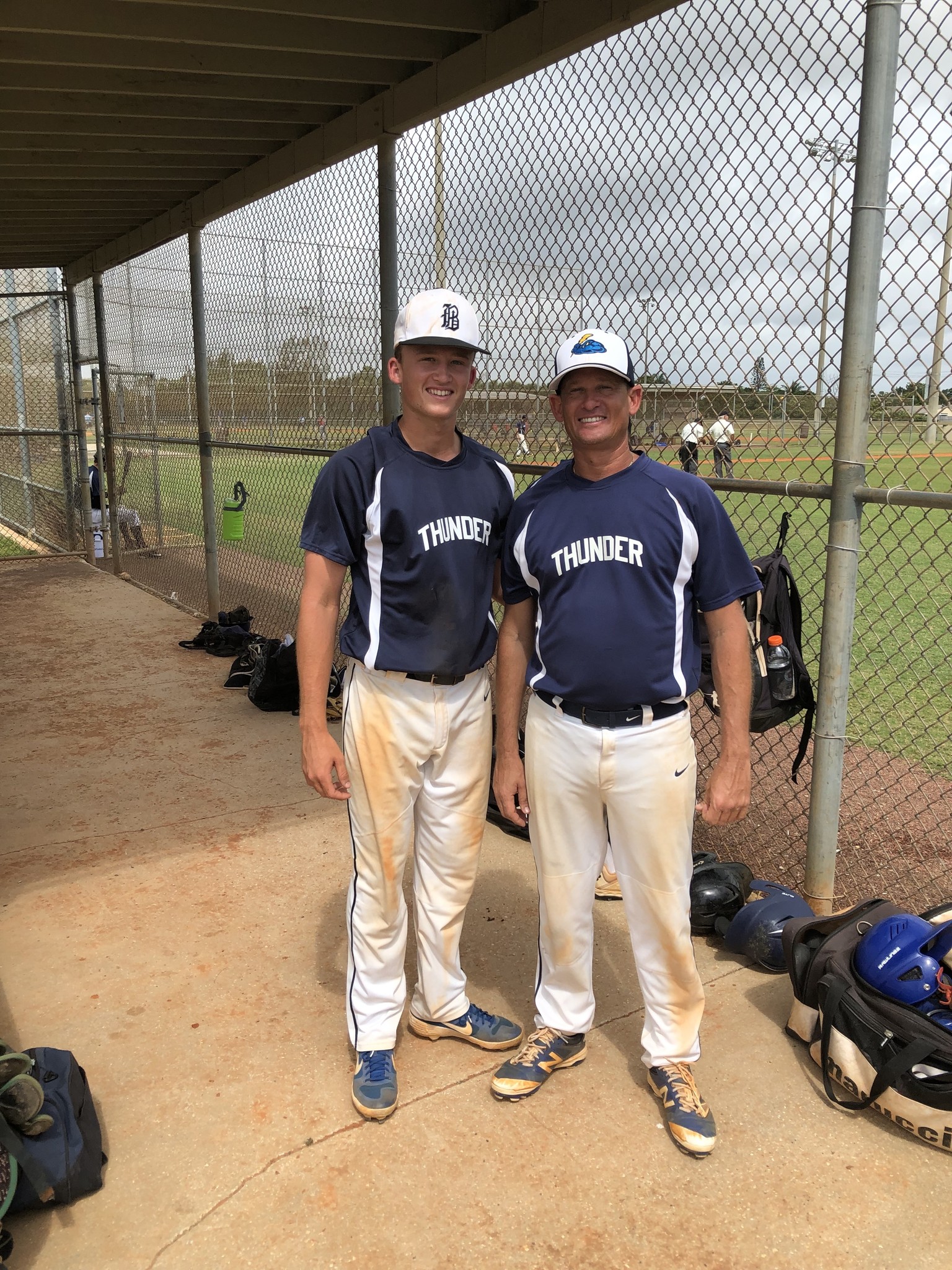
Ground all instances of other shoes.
[137,548,161,559]
[124,540,140,550]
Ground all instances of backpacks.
[695,512,816,785]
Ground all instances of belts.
[540,692,685,727]
[686,441,696,445]
[403,672,468,686]
[718,442,730,446]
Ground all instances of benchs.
[635,436,681,445]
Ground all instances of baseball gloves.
[327,697,344,721]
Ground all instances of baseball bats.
[118,450,133,506]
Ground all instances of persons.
[680,418,706,477]
[294,288,518,1123]
[484,327,753,1161]
[705,410,735,479]
[84,412,93,428]
[516,415,533,459]
[74,447,163,560]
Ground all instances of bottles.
[767,636,795,701]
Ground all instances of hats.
[394,289,491,355]
[694,418,703,426]
[547,328,634,390]
[719,411,729,416]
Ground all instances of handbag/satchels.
[733,439,741,445]
[777,897,952,1155]
[0,1047,108,1219]
[248,638,300,713]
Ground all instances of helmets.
[854,913,951,1004]
[691,861,756,935]
[218,605,253,633]
[94,448,106,468]
[715,879,817,974]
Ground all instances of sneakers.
[527,453,533,457]
[516,455,523,459]
[409,1001,524,1052]
[489,1029,589,1101]
[353,1050,400,1122]
[646,1063,718,1159]
